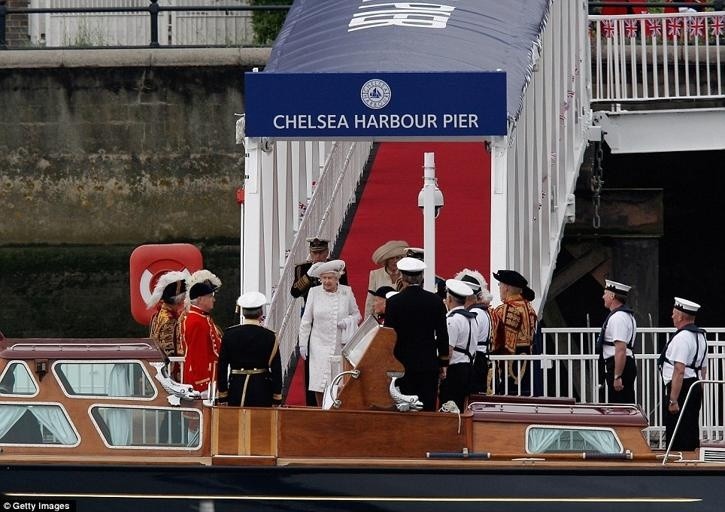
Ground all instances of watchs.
[667,400,678,405]
[613,374,622,379]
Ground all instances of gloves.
[338,321,347,330]
[299,347,308,359]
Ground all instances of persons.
[217,290,283,408]
[659,296,708,450]
[300,260,363,408]
[442,278,479,415]
[453,269,493,394]
[597,278,639,403]
[363,240,409,321]
[181,269,222,399]
[384,256,452,412]
[473,289,508,396]
[291,237,348,406]
[366,285,398,327]
[146,271,190,382]
[404,248,446,299]
[492,270,536,397]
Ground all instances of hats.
[521,288,534,301]
[604,279,632,296]
[146,272,186,308]
[493,271,528,289]
[432,274,443,291]
[444,279,472,302]
[237,293,267,313]
[306,260,345,277]
[404,248,424,258]
[673,297,700,315]
[395,256,424,275]
[371,241,408,265]
[307,237,331,251]
[185,271,221,310]
[454,270,492,304]
[368,286,395,298]
[386,292,400,300]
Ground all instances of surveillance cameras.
[419,188,444,216]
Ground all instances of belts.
[231,368,267,374]
